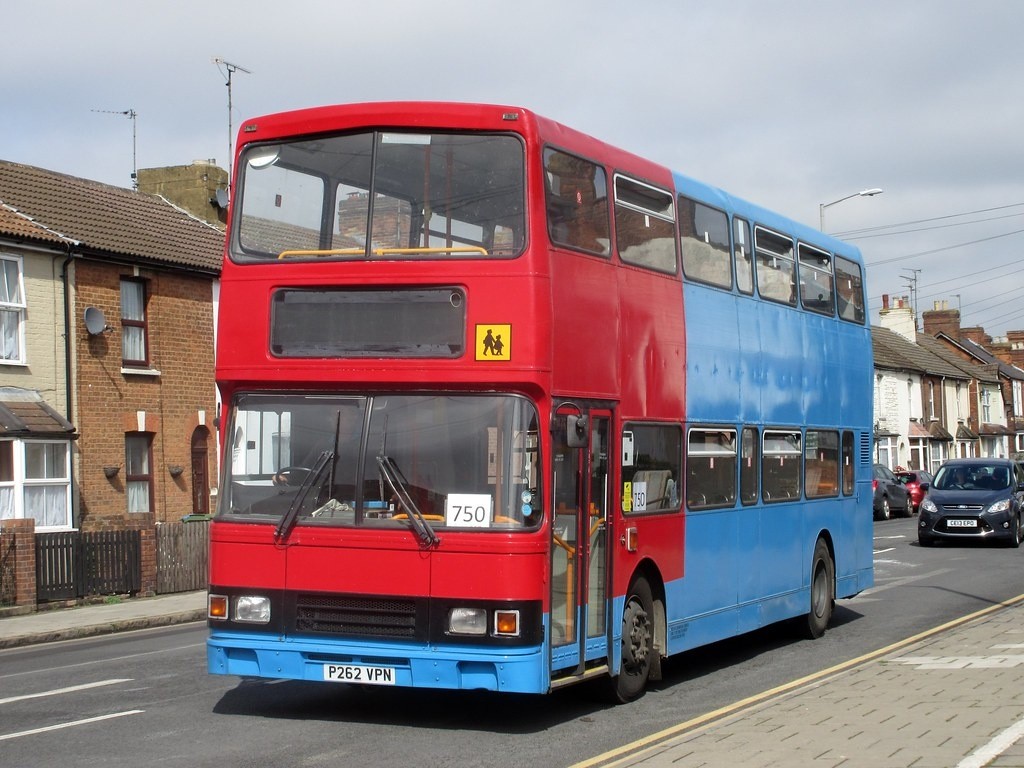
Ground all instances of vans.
[917,458,1024,549]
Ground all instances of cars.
[872,463,914,520]
[893,470,935,513]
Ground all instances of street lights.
[820,188,883,233]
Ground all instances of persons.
[951,469,970,490]
[979,468,1006,488]
[271,399,414,510]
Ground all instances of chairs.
[990,467,1007,489]
[689,485,801,505]
[630,470,675,510]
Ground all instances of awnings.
[1,402,80,439]
[875,419,901,436]
[908,419,953,441]
[956,416,1024,441]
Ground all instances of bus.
[204,101,875,707]
